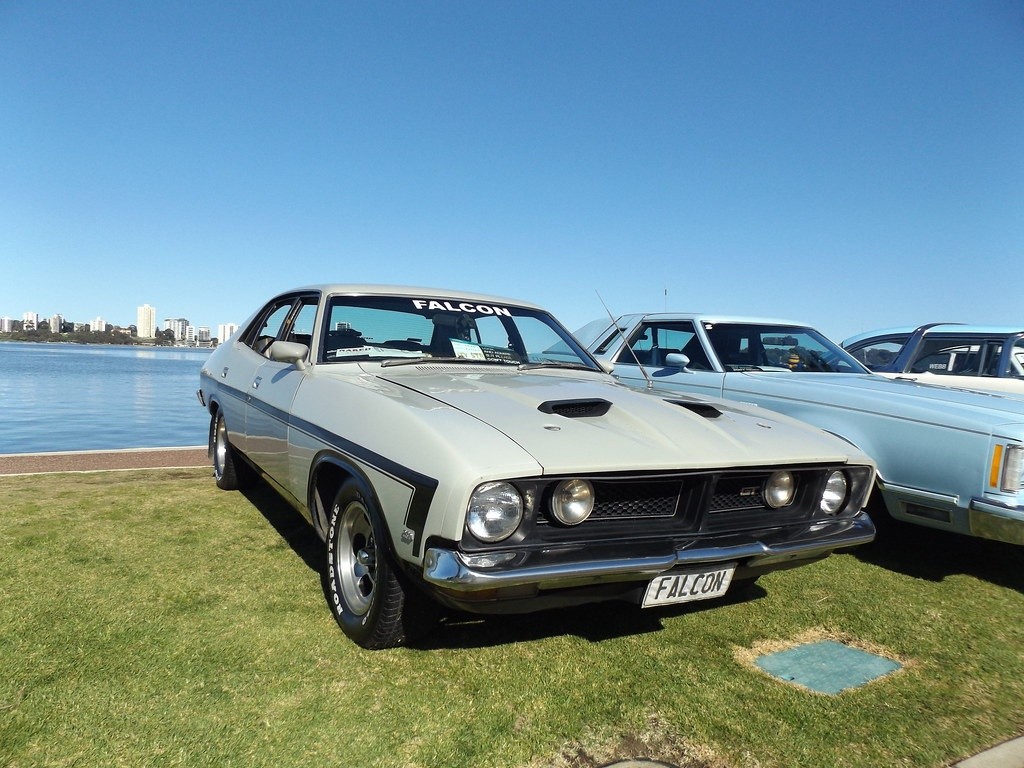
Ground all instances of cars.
[526,309,1024,551]
[818,322,1024,396]
[195,283,879,650]
